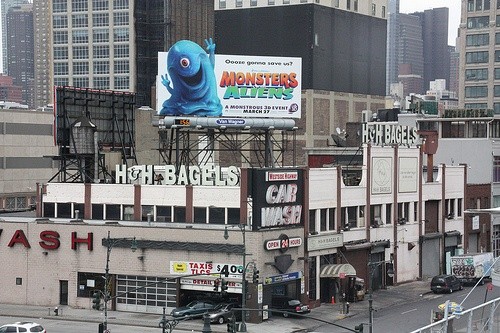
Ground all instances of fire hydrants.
[54,307,59,316]
[346,302,350,314]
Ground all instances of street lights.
[104,231,138,333]
[367,260,395,333]
[224,223,247,333]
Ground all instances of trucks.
[450,252,495,286]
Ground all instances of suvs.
[430,274,463,294]
[170,300,219,320]
[202,302,241,325]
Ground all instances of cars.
[272,297,311,318]
[0,320,47,333]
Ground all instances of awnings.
[320,263,357,277]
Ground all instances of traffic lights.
[92,293,100,310]
[222,280,228,296]
[253,268,260,284]
[213,279,219,292]
